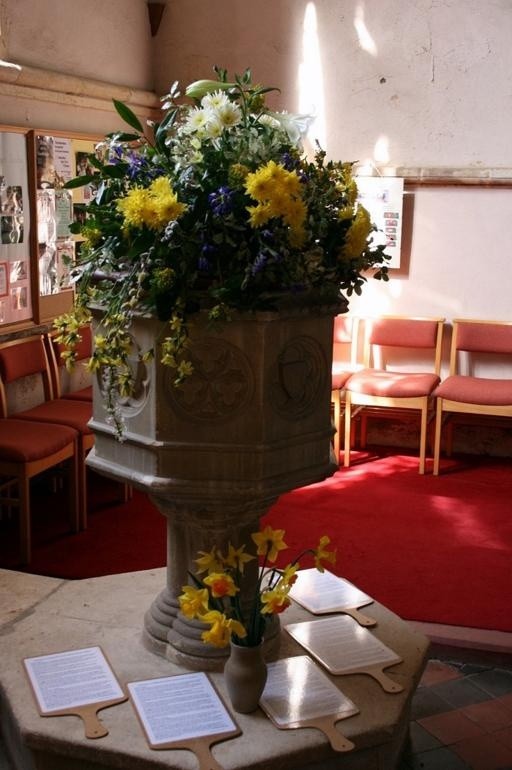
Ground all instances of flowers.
[177,527,336,650]
[47,64,393,447]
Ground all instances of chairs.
[0,323,134,567]
[331,313,511,476]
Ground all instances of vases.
[224,636,268,713]
[82,273,350,672]
